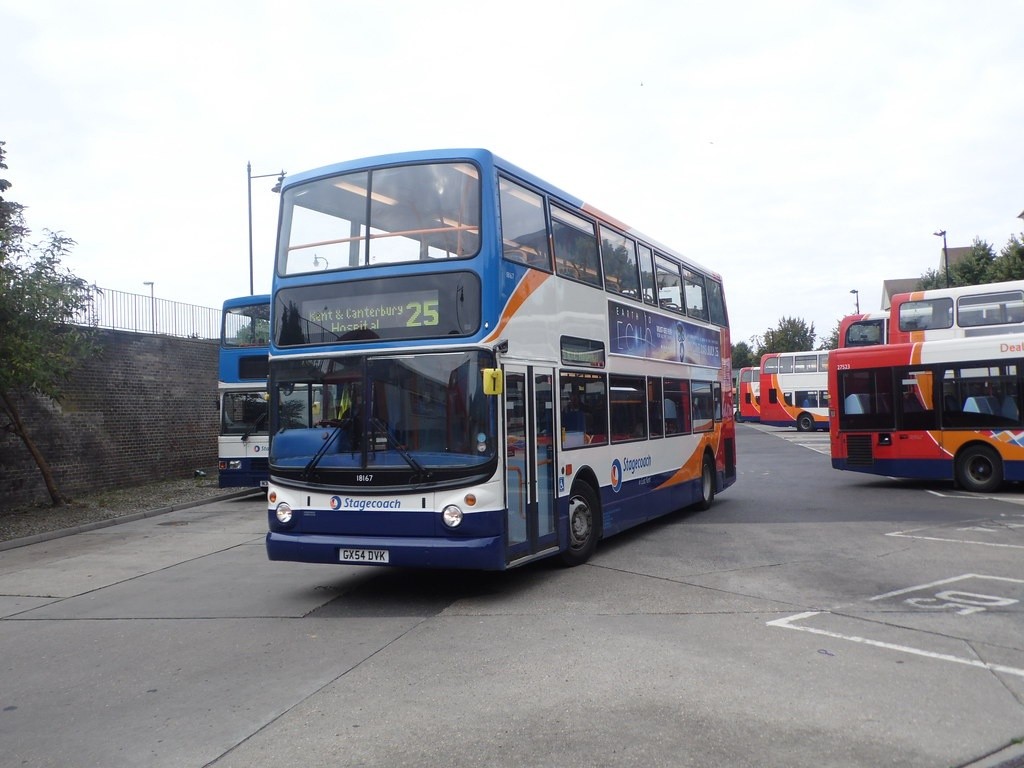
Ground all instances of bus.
[828,280,1024,494]
[265,148,737,576]
[732,388,746,423]
[759,349,829,433]
[217,295,447,493]
[738,364,829,423]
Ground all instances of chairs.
[592,434,630,444]
[845,391,1019,422]
[504,249,708,320]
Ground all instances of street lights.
[143,281,155,333]
[851,289,860,314]
[932,229,951,290]
[245,161,286,344]
